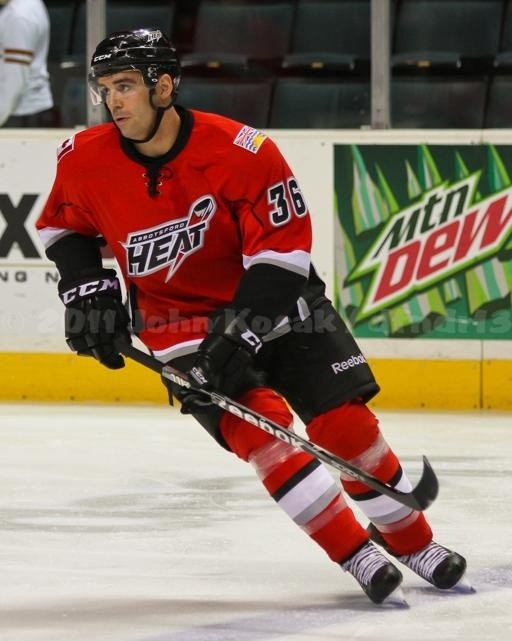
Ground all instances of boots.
[339,539,401,602]
[367,523,466,589]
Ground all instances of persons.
[35,25,467,604]
[0,0,56,127]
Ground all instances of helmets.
[86,27,181,93]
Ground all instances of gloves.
[181,305,276,416]
[57,267,132,369]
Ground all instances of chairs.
[44,1,512,129]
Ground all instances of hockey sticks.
[124,344,440,511]
